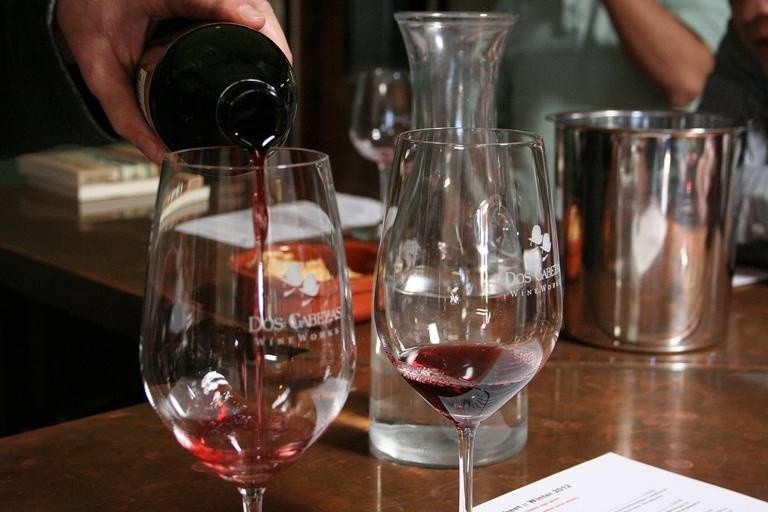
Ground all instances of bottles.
[134,24,300,173]
[369,10,531,467]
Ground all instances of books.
[159,171,211,233]
[16,143,160,202]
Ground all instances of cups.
[546,109,754,354]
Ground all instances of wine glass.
[369,129,560,512]
[137,146,358,512]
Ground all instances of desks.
[0,249,768,512]
[1,153,376,371]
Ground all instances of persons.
[0,0,294,171]
[695,0,768,273]
[493,0,734,226]
